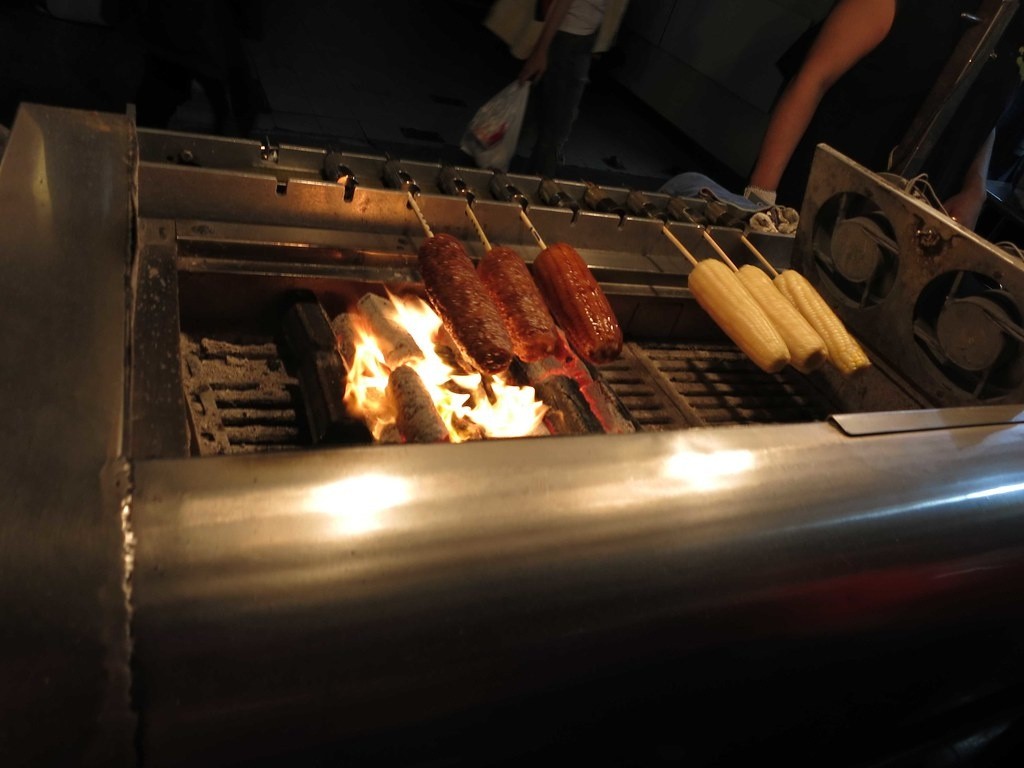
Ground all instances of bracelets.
[743,187,778,207]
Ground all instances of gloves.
[743,185,778,206]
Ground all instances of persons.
[743,0,1024,241]
[485,1,629,180]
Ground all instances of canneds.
[469,117,508,150]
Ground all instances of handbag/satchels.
[460,79,531,173]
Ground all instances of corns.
[417,234,558,375]
[688,258,871,382]
[534,243,622,363]
